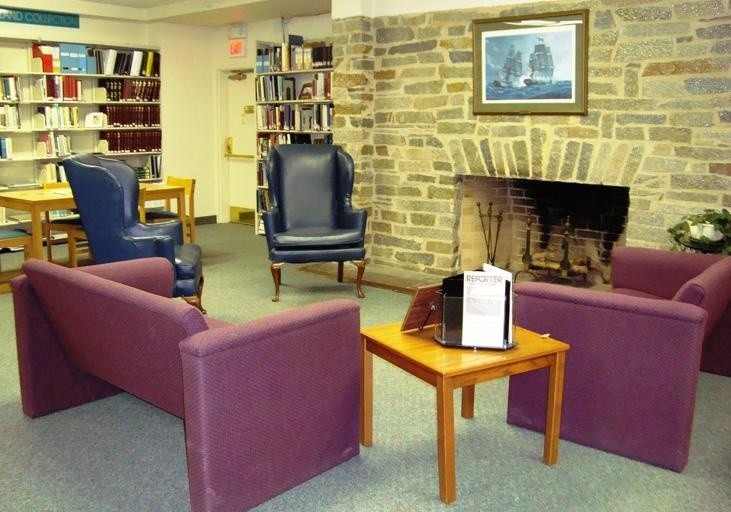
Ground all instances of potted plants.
[667,208,731,256]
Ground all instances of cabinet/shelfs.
[1,35,165,252]
[255,35,335,235]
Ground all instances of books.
[256,33,334,209]
[1,39,163,218]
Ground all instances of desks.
[361,320,571,504]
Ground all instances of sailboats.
[522,36,554,86]
[493,43,523,88]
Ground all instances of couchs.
[9,255,362,511]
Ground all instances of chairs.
[56,151,207,314]
[506,245,731,472]
[262,143,368,301]
[0,175,196,292]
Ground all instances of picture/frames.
[399,283,444,332]
[471,9,589,115]
